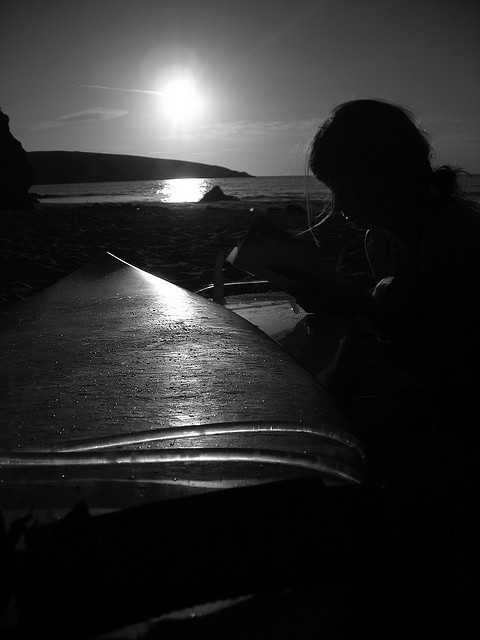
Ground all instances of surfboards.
[3,248,372,526]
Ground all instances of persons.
[280,100,479,402]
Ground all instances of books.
[226,210,350,302]
